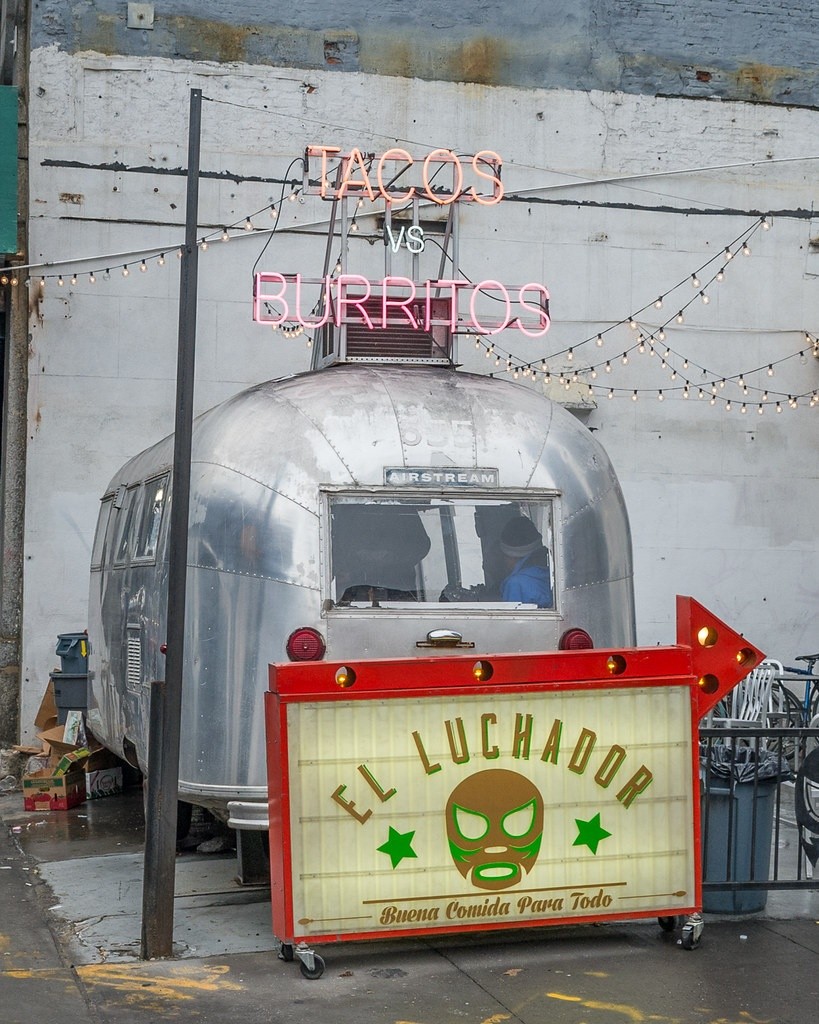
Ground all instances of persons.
[498,517,551,607]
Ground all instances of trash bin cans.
[700,741,794,918]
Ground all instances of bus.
[85,358,638,891]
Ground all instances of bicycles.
[692,652,819,787]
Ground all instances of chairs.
[699,660,799,752]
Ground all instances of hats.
[499,516,544,558]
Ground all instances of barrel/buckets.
[48,671,87,725]
[55,633,88,673]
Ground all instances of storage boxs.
[49,671,89,707]
[20,707,123,810]
[55,631,88,673]
